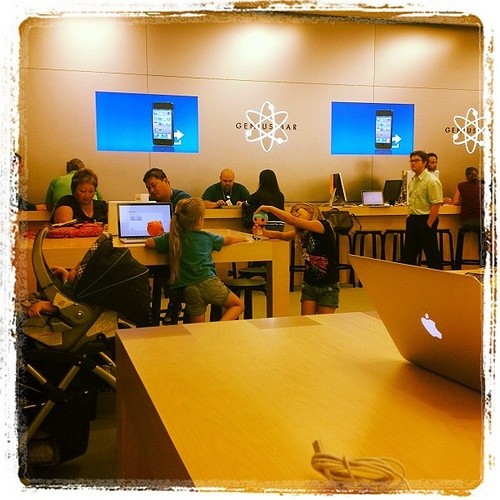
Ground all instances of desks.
[114,311,483,486]
[30,228,290,319]
[203,205,478,284]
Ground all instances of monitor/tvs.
[329,174,348,208]
[383,179,403,205]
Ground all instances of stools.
[223,268,272,320]
[289,228,480,292]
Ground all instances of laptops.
[347,254,482,392]
[108,201,173,244]
[361,191,389,208]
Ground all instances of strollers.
[15,227,154,479]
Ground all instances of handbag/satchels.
[321,208,353,232]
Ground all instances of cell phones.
[375,109,393,149]
[152,101,174,146]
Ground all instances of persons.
[145,197,249,324]
[11,153,47,211]
[142,168,192,326]
[26,260,83,318]
[401,150,444,270]
[252,201,341,316]
[241,169,285,269]
[426,152,438,172]
[202,168,250,209]
[453,167,485,229]
[45,158,105,214]
[50,168,109,228]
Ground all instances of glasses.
[409,159,423,164]
